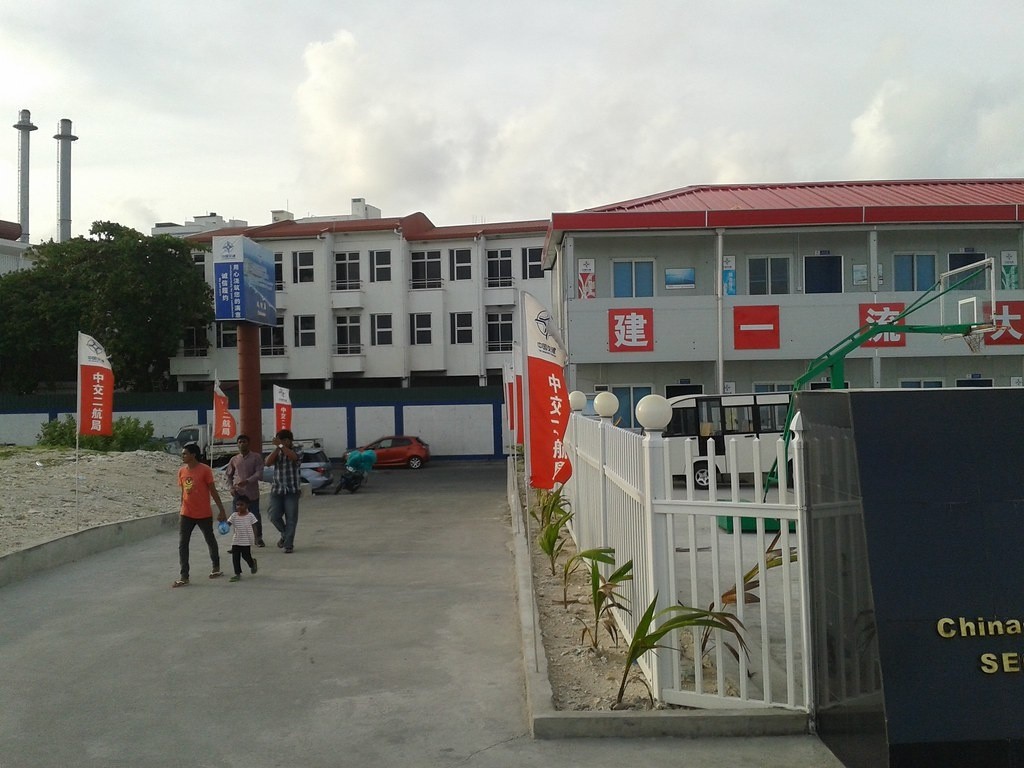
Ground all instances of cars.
[346,436,430,470]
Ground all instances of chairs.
[741,420,755,432]
[699,421,714,436]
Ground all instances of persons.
[217,495,258,582]
[225,434,264,554]
[264,429,304,553]
[171,444,227,588]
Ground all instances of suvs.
[219,447,334,495]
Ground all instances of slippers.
[172,580,189,588]
[209,570,224,578]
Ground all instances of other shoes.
[277,538,285,548]
[283,548,293,553]
[251,558,258,574]
[227,549,232,554]
[229,575,241,582]
[256,541,265,547]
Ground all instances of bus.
[641,390,800,490]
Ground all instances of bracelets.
[278,443,284,449]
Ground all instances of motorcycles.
[334,463,364,494]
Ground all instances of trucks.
[164,423,323,468]
